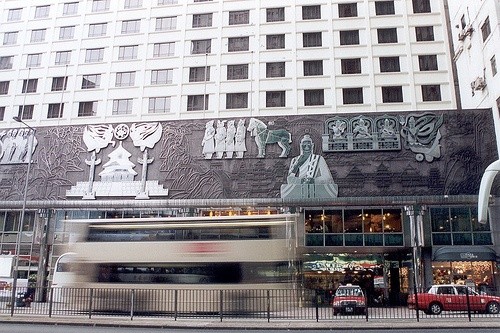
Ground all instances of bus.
[64,213,300,315]
[48,253,76,302]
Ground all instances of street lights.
[10,117,37,317]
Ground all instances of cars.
[407,284,499,314]
[333,283,365,315]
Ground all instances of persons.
[0,128,39,164]
[201,118,246,160]
[281,132,338,198]
